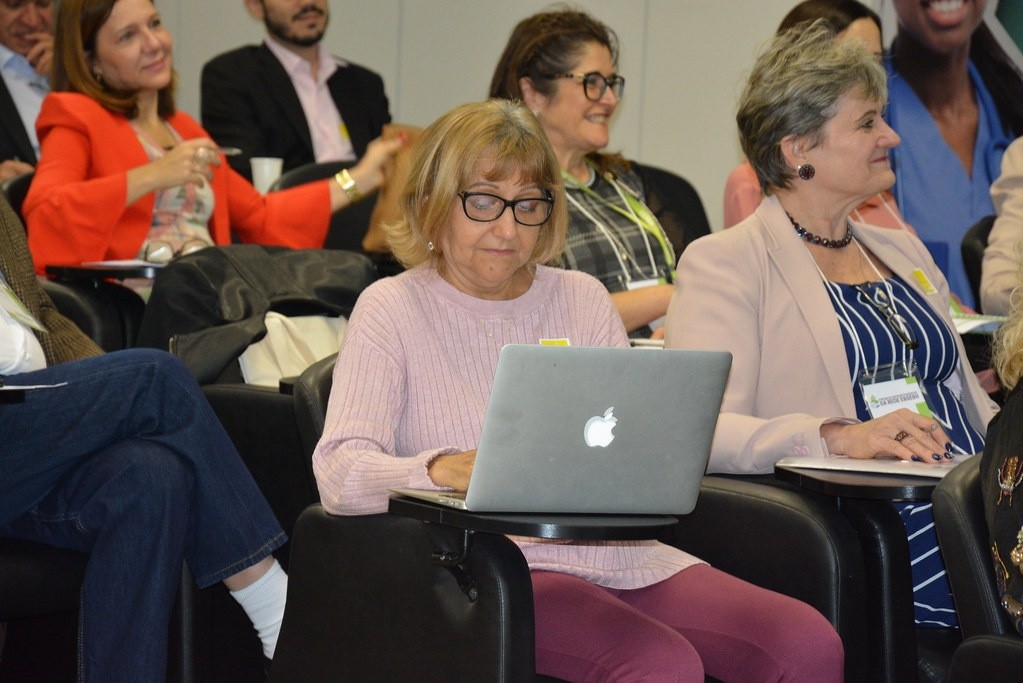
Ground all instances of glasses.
[456,189,555,226]
[560,72,625,104]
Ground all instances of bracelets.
[336,169,360,203]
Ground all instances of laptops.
[388,344,734,513]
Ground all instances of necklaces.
[788,212,853,248]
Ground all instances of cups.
[250,157,283,196]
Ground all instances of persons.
[201,0,392,193]
[723,0,1023,632]
[880,0,1023,316]
[490,11,679,343]
[313,99,845,683]
[666,25,1003,634]
[0,194,288,683]
[21,0,403,299]
[0,0,58,184]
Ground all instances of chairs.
[0,160,1023,683]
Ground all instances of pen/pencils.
[926,424,937,431]
[162,144,243,156]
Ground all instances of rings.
[196,147,207,159]
[895,431,908,441]
